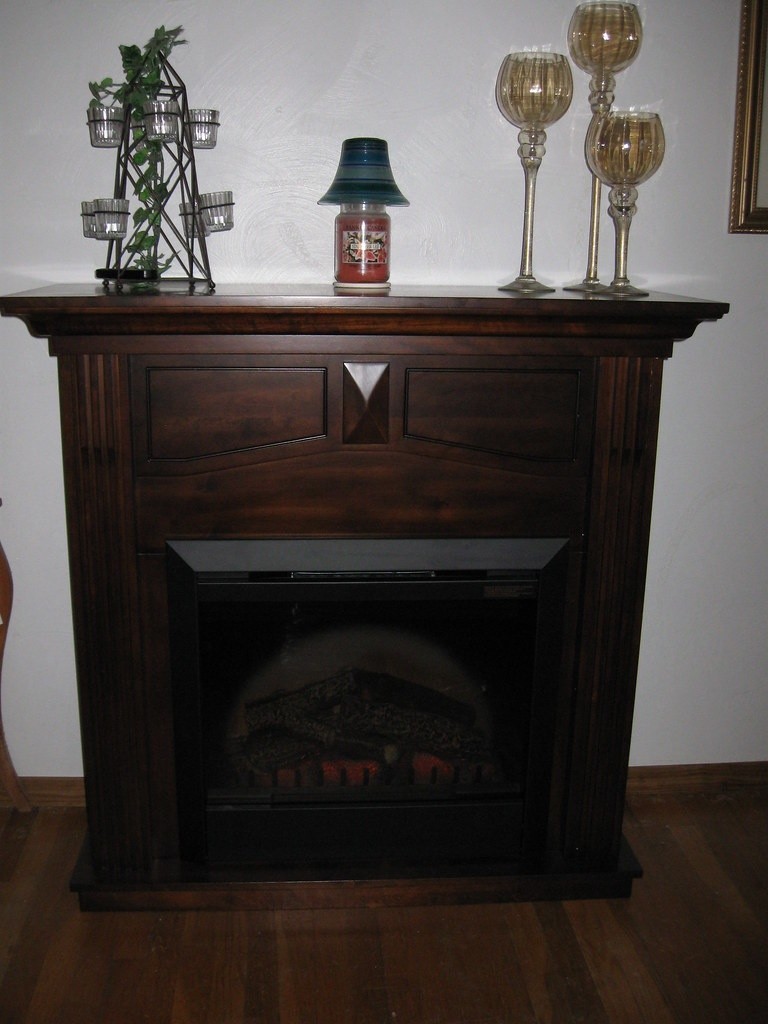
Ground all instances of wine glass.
[584,110,665,297]
[495,52,574,293]
[563,1,642,294]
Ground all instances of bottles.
[333,203,391,283]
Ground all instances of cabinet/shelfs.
[0,287,730,914]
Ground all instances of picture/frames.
[728,0,768,235]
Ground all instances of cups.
[86,107,124,147]
[93,199,128,239]
[200,191,234,231]
[141,102,177,142]
[180,203,212,239]
[185,109,219,149]
[317,138,410,207]
[81,202,96,238]
